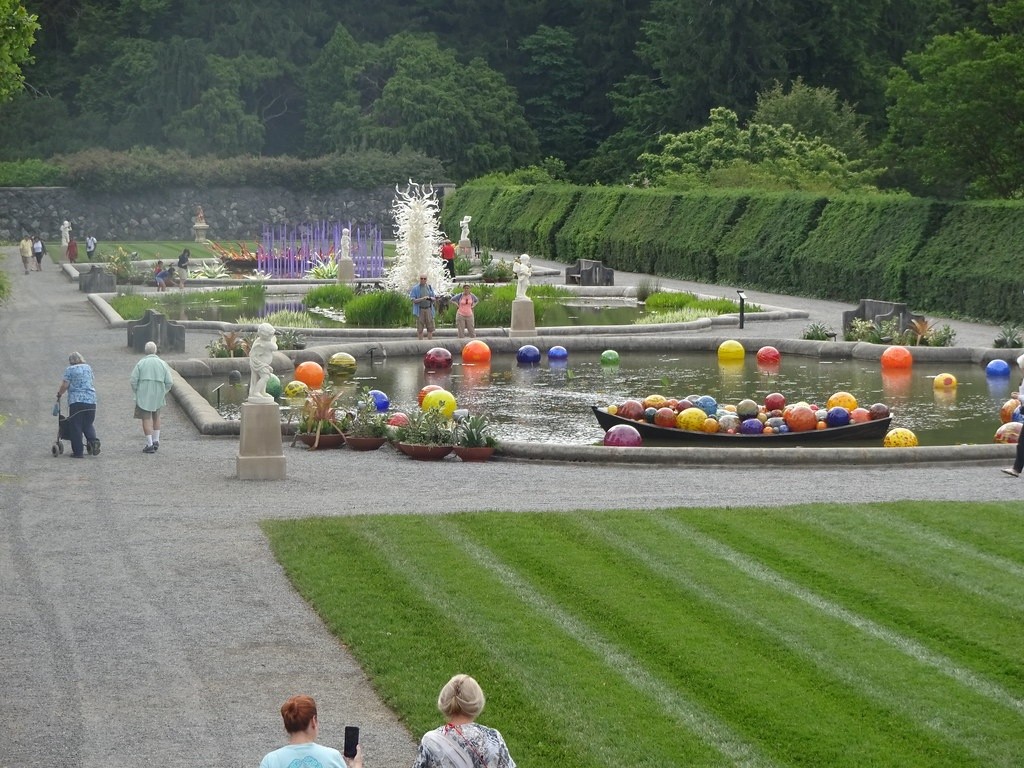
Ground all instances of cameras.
[426,298,431,300]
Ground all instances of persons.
[131,341,173,452]
[1000,353,1024,478]
[513,254,531,299]
[60,221,72,243]
[411,274,435,340]
[20,235,33,274]
[33,236,48,270]
[412,674,518,768]
[156,267,175,292]
[178,249,189,288]
[258,695,363,768]
[155,261,163,274]
[56,350,101,457]
[66,237,78,263]
[449,284,479,339]
[460,214,472,240]
[249,323,278,397]
[442,239,455,282]
[86,232,97,259]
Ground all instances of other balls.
[367,384,458,428]
[932,372,957,388]
[228,351,358,398]
[516,344,568,363]
[424,339,491,369]
[994,398,1024,443]
[600,349,621,366]
[717,339,781,364]
[985,359,1011,377]
[880,346,913,369]
[603,391,919,448]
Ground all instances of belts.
[419,306,431,309]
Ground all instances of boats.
[590,404,892,449]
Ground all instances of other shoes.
[1001,468,1020,477]
[143,445,155,453]
[153,441,159,450]
[70,450,84,458]
[93,440,101,455]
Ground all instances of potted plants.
[291,331,307,350]
[205,330,248,358]
[398,401,453,460]
[295,381,351,449]
[345,385,388,450]
[393,408,422,452]
[454,410,498,462]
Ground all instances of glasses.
[466,299,468,304]
[421,278,427,280]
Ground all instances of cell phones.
[344,726,359,758]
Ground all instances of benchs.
[842,299,926,342]
[565,259,614,285]
[79,265,116,294]
[128,309,185,354]
